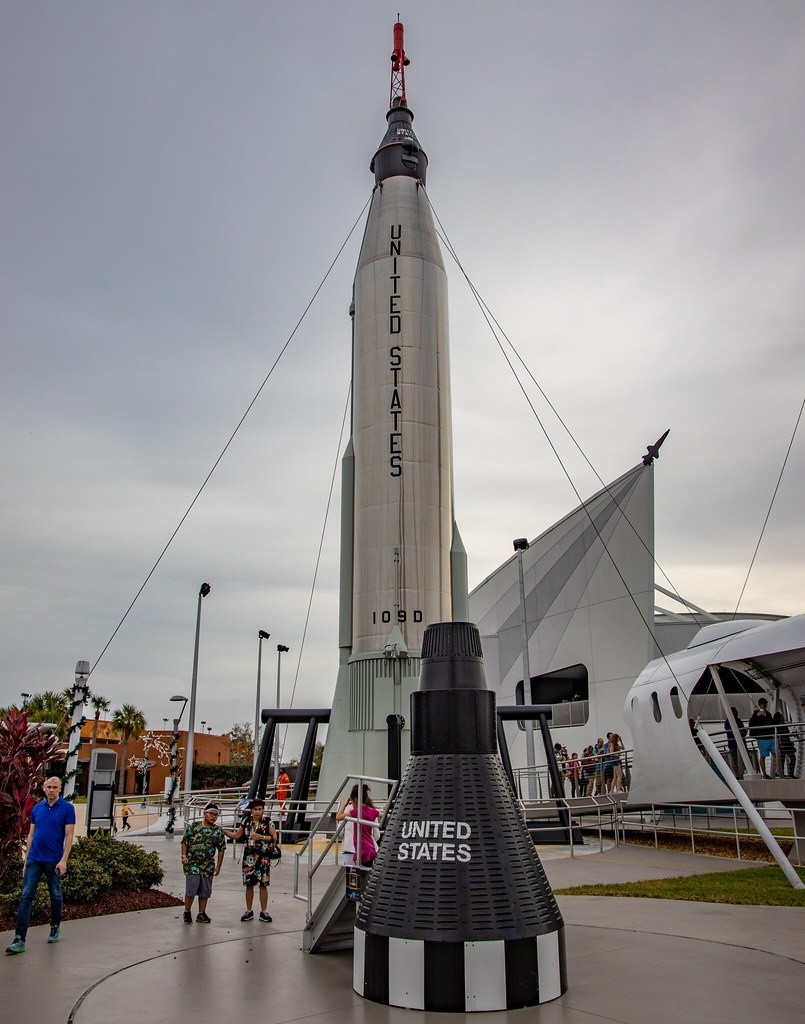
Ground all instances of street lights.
[510,537,541,804]
[207,726,212,733]
[163,694,189,840]
[102,708,111,720]
[162,718,169,730]
[182,581,214,819]
[252,629,272,776]
[201,721,207,733]
[274,644,291,783]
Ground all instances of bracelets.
[263,836,266,840]
[182,855,186,858]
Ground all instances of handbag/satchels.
[267,837,281,859]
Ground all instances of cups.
[760,709,763,714]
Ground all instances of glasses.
[205,810,218,817]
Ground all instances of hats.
[204,803,219,813]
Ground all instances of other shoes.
[787,773,799,779]
[278,813,285,816]
[616,790,624,793]
[736,776,740,780]
[762,774,773,780]
[127,825,131,830]
[775,773,788,778]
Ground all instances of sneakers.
[259,911,272,922]
[240,911,254,921]
[183,910,192,923]
[5,934,26,953]
[196,912,211,923]
[47,924,61,943]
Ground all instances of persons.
[111,815,118,834]
[773,711,799,779]
[180,803,226,923]
[218,798,277,922]
[749,698,788,779]
[337,784,390,899]
[690,719,705,755]
[5,776,75,952]
[724,707,747,776]
[277,767,290,816]
[550,732,624,798]
[121,799,134,831]
